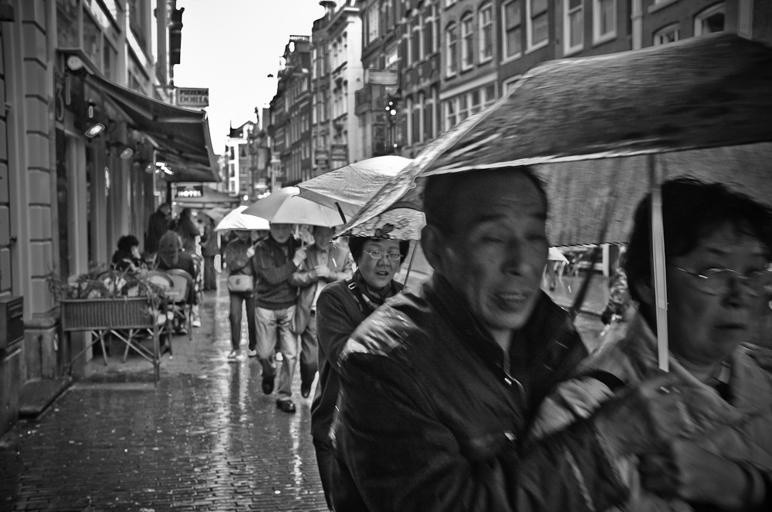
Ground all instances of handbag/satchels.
[227,240,257,293]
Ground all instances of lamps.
[118,143,136,161]
[131,159,142,169]
[144,161,154,174]
[82,119,105,138]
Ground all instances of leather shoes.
[275,398,295,412]
[300,380,313,398]
[262,372,276,394]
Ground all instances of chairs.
[123,272,173,361]
[190,253,204,301]
[167,268,195,342]
[87,271,131,365]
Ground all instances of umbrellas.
[240,185,351,228]
[331,32,772,248]
[213,204,271,232]
[293,154,412,217]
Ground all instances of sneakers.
[228,349,240,358]
[275,352,283,361]
[248,349,258,357]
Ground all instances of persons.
[225,230,255,358]
[249,224,307,412]
[146,203,170,252]
[289,225,355,397]
[309,237,409,508]
[158,230,195,335]
[111,235,149,272]
[327,162,680,512]
[524,176,772,512]
[198,216,217,290]
[177,206,199,254]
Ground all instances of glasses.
[361,249,404,261]
[666,257,772,298]
